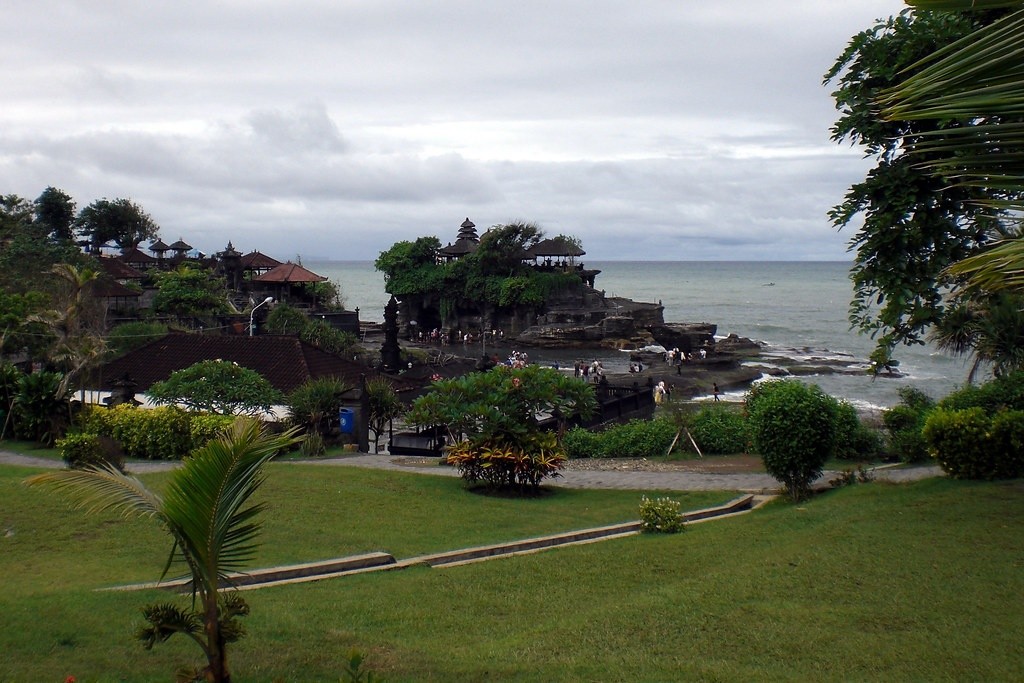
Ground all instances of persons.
[662,348,706,367]
[474,349,528,371]
[459,323,503,346]
[629,363,643,373]
[647,324,654,334]
[574,358,606,383]
[711,382,719,401]
[652,381,675,407]
[553,360,560,370]
[607,339,624,350]
[409,328,448,346]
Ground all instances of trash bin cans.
[339,406,356,433]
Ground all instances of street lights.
[250,297,272,337]
